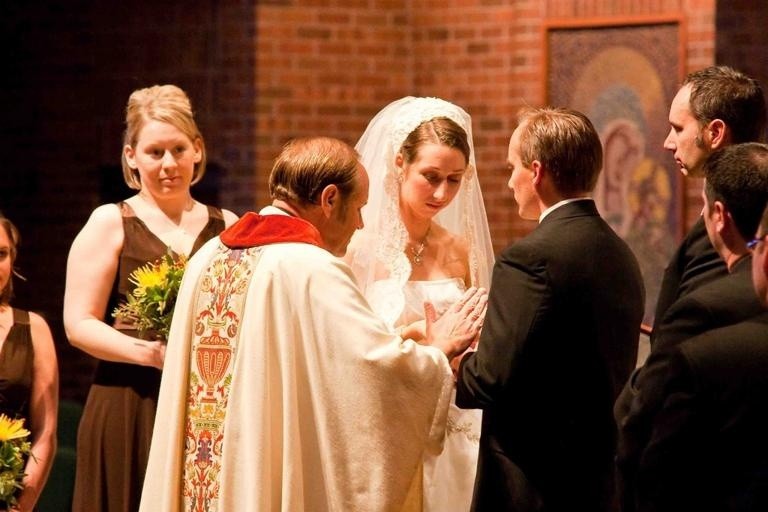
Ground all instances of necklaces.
[394,221,431,266]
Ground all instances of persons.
[630,204,766,508]
[447,103,646,512]
[1,216,59,510]
[62,82,239,512]
[349,93,496,512]
[650,65,768,344]
[613,142,767,435]
[135,139,488,511]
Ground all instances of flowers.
[111,247,187,342]
[0,413,42,512]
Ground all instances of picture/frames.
[540,13,689,336]
